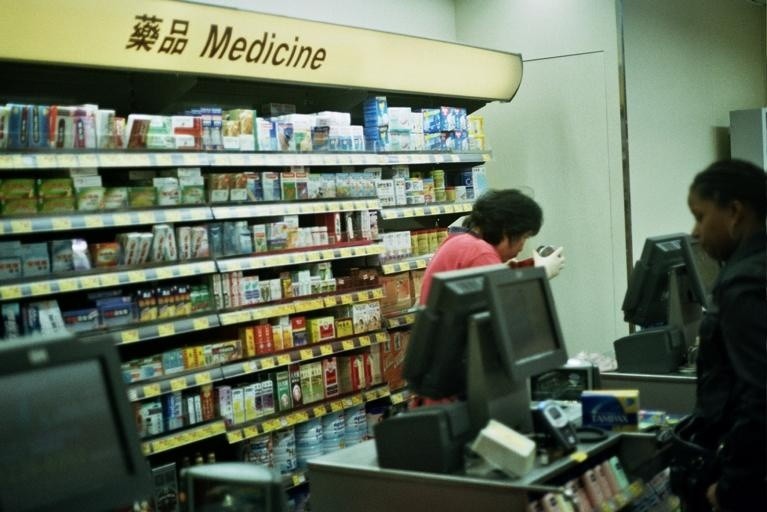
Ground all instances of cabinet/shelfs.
[0,0,526,462]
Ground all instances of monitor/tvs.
[621,232,688,328]
[0,334,155,512]
[483,265,567,382]
[401,263,510,399]
[679,234,723,310]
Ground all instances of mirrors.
[614,0,766,333]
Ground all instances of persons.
[409,188,566,410]
[659,158,767,512]
